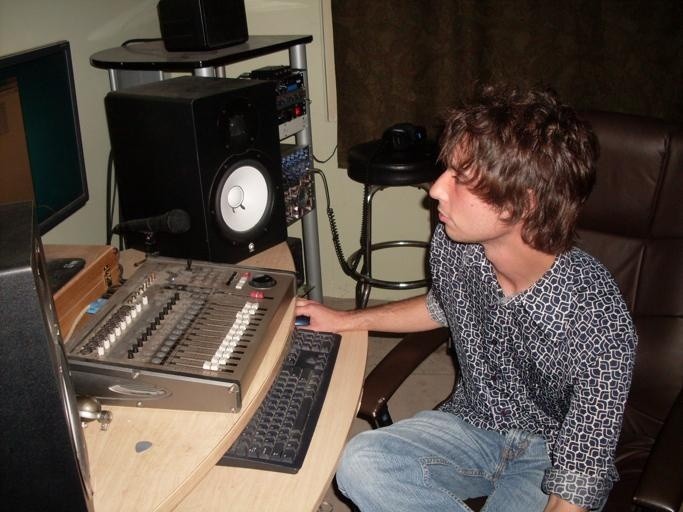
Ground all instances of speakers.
[157,0,249,53]
[1,201,96,512]
[103,75,288,264]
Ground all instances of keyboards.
[216,329,342,475]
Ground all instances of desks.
[73,243,368,512]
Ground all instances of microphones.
[112,210,191,238]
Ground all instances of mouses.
[294,315,309,326]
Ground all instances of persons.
[293,71,639,511]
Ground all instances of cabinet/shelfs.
[89,32,323,305]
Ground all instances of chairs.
[357,106,683,512]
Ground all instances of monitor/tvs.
[0,39,90,293]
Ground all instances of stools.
[345,119,453,356]
